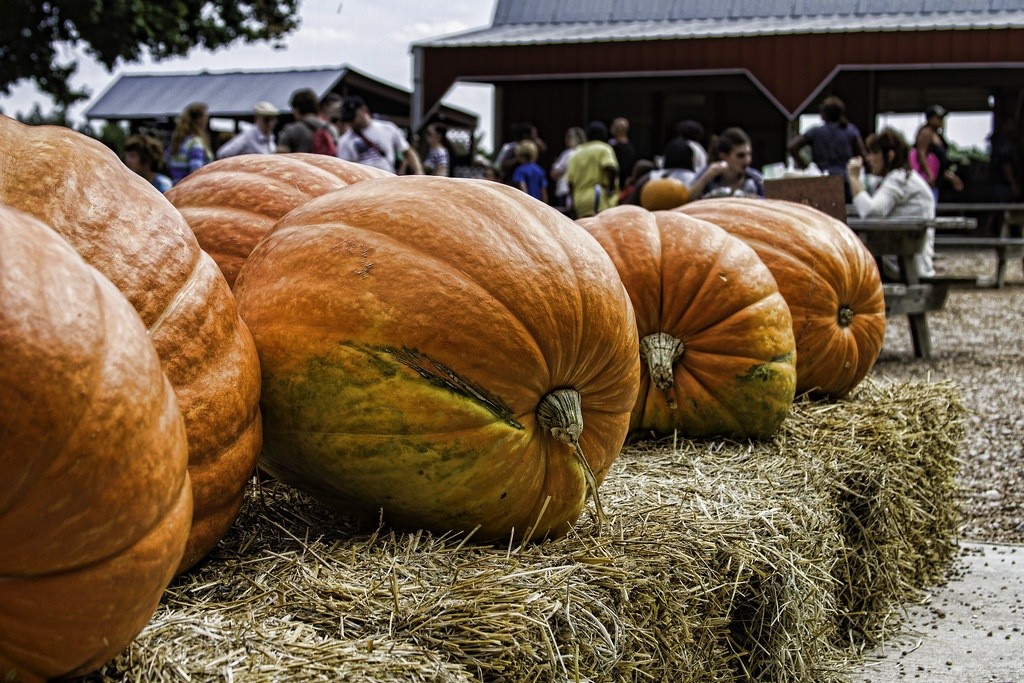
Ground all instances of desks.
[934,203,1024,289]
[850,214,977,356]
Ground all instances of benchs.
[883,274,991,312]
[934,236,1024,246]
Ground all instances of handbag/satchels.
[908,146,940,183]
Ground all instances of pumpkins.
[0,120,887,683]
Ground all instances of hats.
[341,96,364,121]
[252,99,281,116]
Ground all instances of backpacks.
[296,117,338,156]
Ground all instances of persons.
[122,87,974,276]
